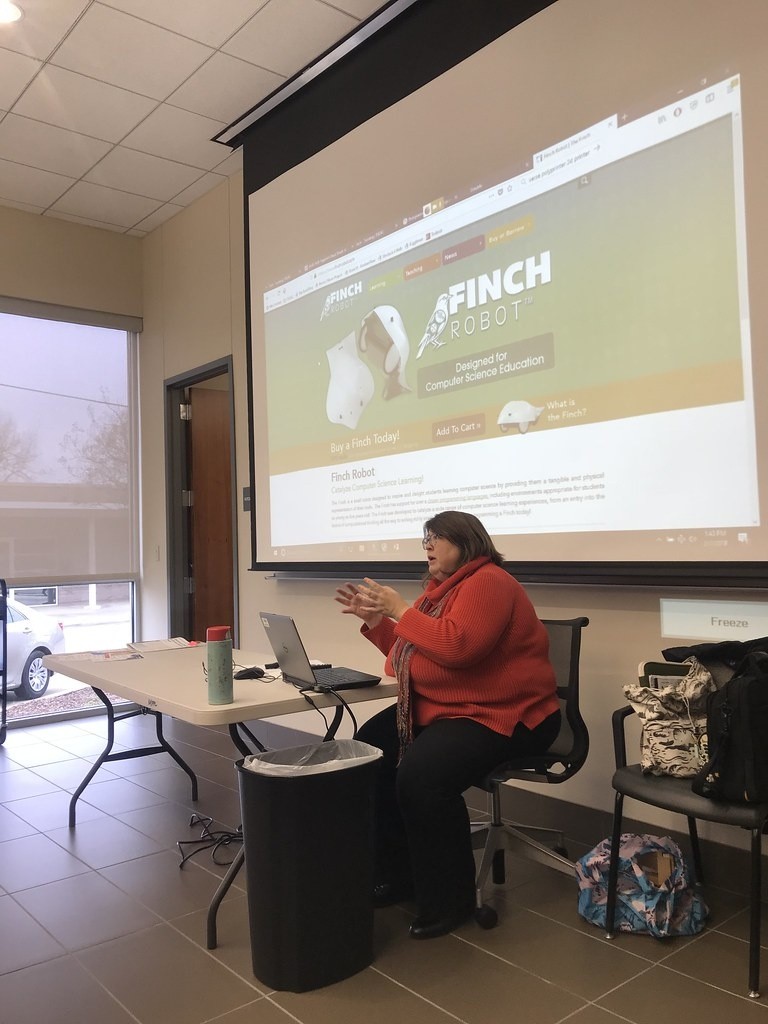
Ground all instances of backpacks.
[690,651,768,805]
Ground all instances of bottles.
[206,626,234,706]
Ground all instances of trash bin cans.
[232,738,385,993]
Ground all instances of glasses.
[422,533,448,550]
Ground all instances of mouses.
[233,667,265,680]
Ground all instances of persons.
[336,511,562,939]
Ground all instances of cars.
[0,595,66,700]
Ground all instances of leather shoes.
[371,880,414,907]
[409,906,473,939]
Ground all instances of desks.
[42,638,399,950]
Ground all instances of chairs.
[470,617,590,931]
[605,661,768,998]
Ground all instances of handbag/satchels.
[621,653,716,777]
[576,833,709,939]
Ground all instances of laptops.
[259,612,382,694]
